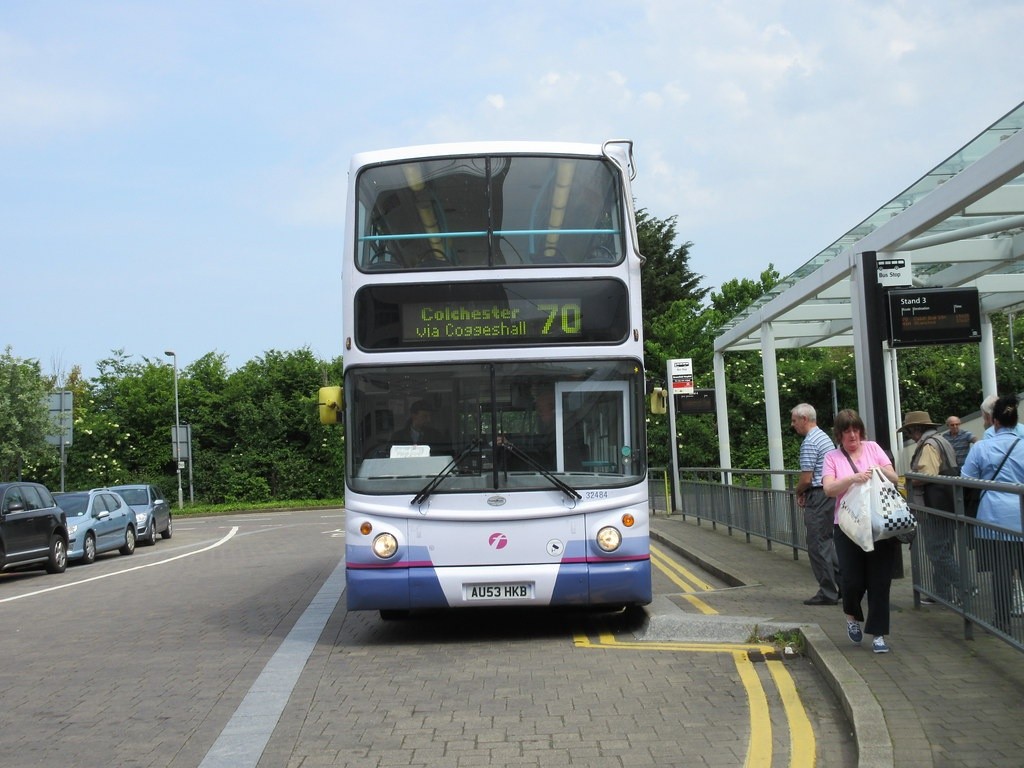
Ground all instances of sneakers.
[847,619,863,643]
[872,635,890,653]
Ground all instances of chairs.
[538,245,569,264]
[364,250,403,270]
[580,244,617,263]
[415,248,455,267]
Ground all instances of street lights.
[165,351,183,510]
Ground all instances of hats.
[410,401,438,414]
[897,411,941,434]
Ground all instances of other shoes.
[952,585,977,606]
[804,589,837,605]
[920,597,955,605]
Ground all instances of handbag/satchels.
[837,478,874,553]
[871,467,917,542]
[897,529,917,549]
[917,436,969,512]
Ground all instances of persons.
[497,389,582,472]
[895,397,1024,644]
[386,401,445,453]
[820,409,897,654]
[791,404,842,605]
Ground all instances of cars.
[109,483,173,545]
[52,487,138,564]
[0,482,69,573]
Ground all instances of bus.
[317,140,671,621]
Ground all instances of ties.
[416,432,424,445]
